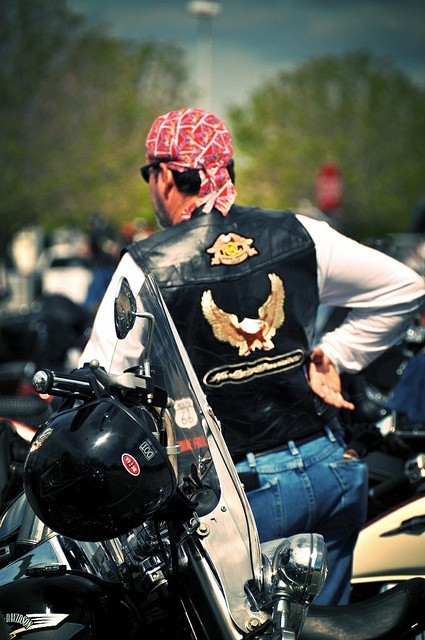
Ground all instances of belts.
[253,433,328,458]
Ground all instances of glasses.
[140,157,178,183]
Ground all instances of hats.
[144,107,238,222]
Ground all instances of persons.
[78,105,425,608]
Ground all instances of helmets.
[22,398,178,542]
